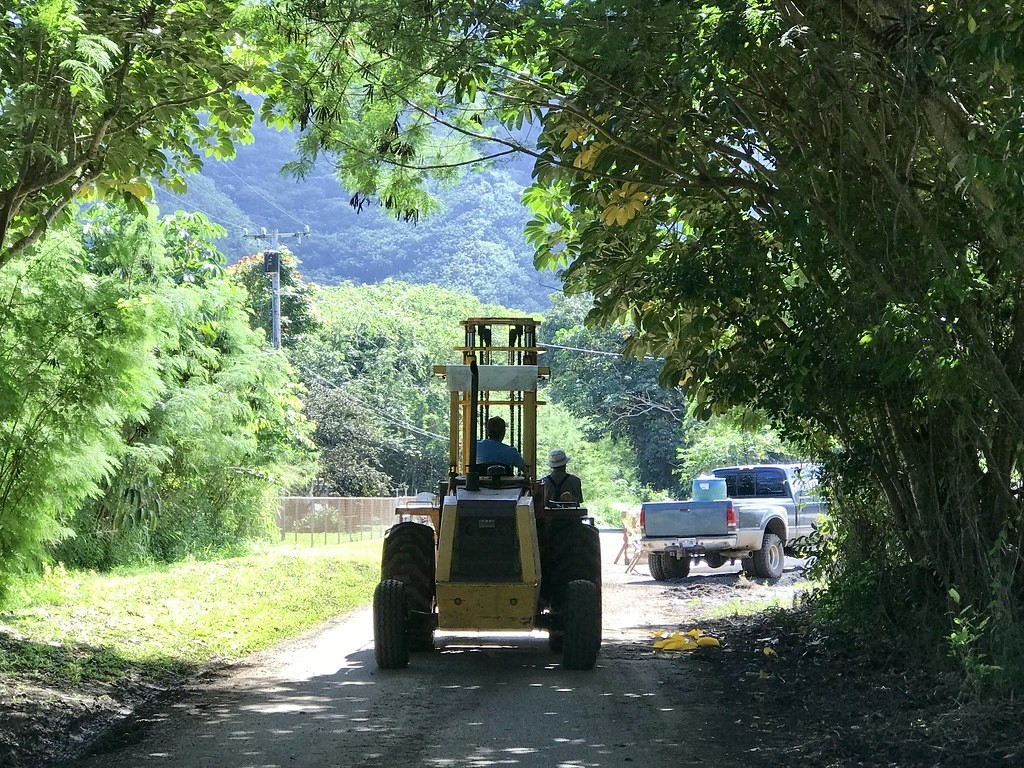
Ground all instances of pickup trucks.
[633,461,840,578]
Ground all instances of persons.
[476,416,525,473]
[539,451,584,507]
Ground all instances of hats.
[487,416,506,433]
[546,450,571,467]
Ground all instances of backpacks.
[547,474,571,507]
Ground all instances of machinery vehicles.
[372,319,602,669]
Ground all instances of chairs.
[477,463,514,477]
